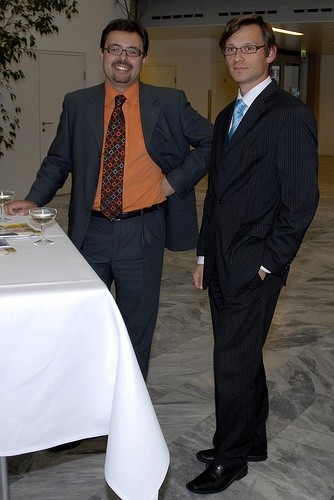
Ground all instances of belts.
[92,200,167,221]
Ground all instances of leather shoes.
[196,445,267,463]
[185,460,248,493]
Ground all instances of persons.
[4,18,214,451]
[186,14,320,495]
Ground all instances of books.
[0,223,41,255]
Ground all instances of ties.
[98,94,127,223]
[228,98,248,141]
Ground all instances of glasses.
[103,45,145,58]
[222,45,266,56]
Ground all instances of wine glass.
[29,207,57,246]
[0,186,16,223]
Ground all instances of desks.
[0,203,171,500]
